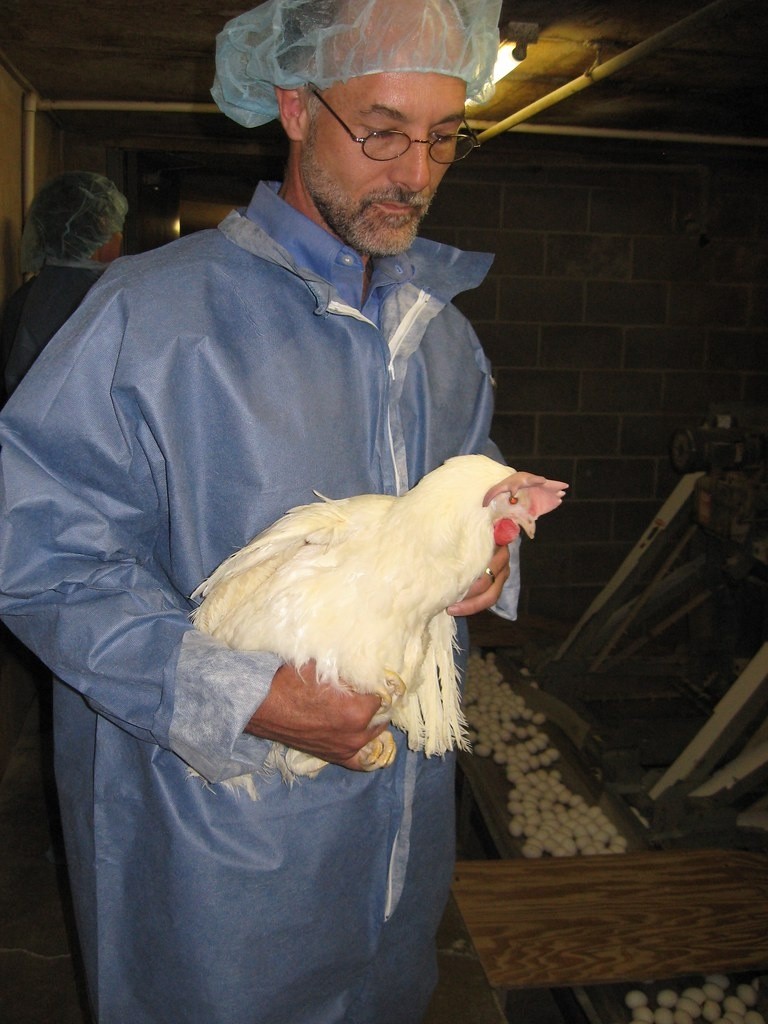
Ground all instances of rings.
[485,568,495,583]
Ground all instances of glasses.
[310,85,481,164]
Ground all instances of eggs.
[459,651,649,859]
[624,973,768,1024]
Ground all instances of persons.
[0,0,520,1024]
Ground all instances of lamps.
[465,22,540,106]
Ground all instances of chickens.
[182,454,570,802]
